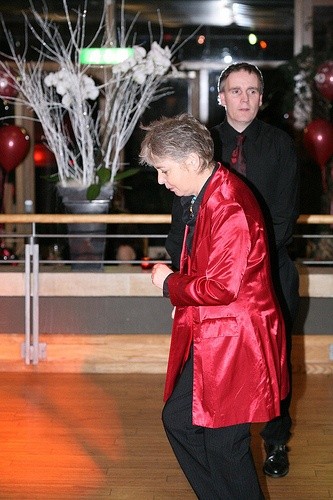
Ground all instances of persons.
[166,63,300,477]
[139,112,289,500]
[105,190,145,267]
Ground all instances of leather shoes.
[262,443,289,478]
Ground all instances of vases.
[57,181,113,273]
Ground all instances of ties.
[230,134,246,178]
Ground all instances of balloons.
[303,119,333,165]
[0,69,19,101]
[0,125,31,173]
[314,60,333,103]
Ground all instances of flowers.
[1,0,202,203]
